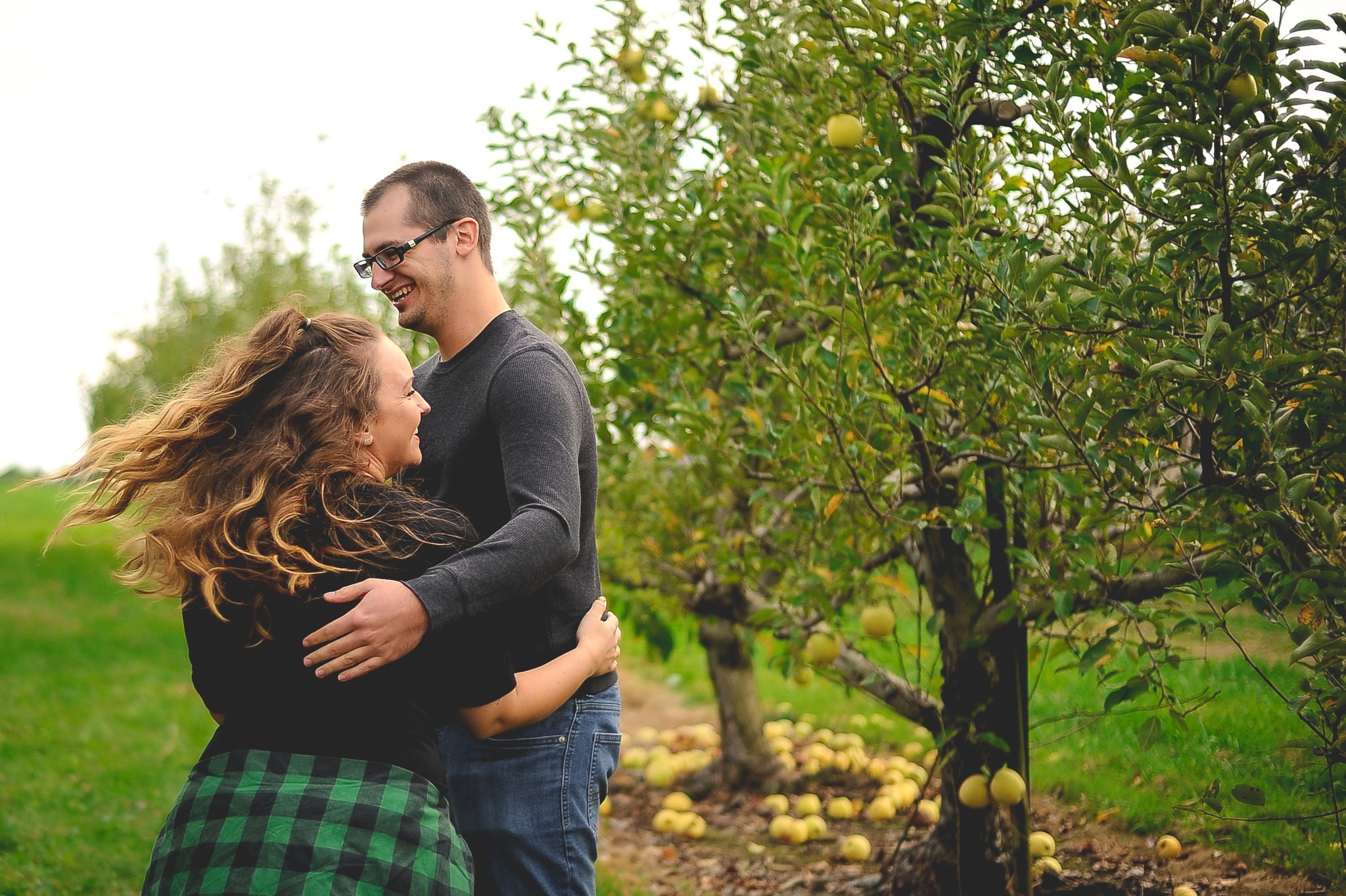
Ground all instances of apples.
[599,701,1200,896]
[793,631,841,685]
[551,196,606,223]
[861,604,895,636]
[1228,16,1273,103]
[617,47,719,122]
[826,114,863,148]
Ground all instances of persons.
[303,161,620,896]
[18,306,621,896]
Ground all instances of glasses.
[353,219,461,278]
